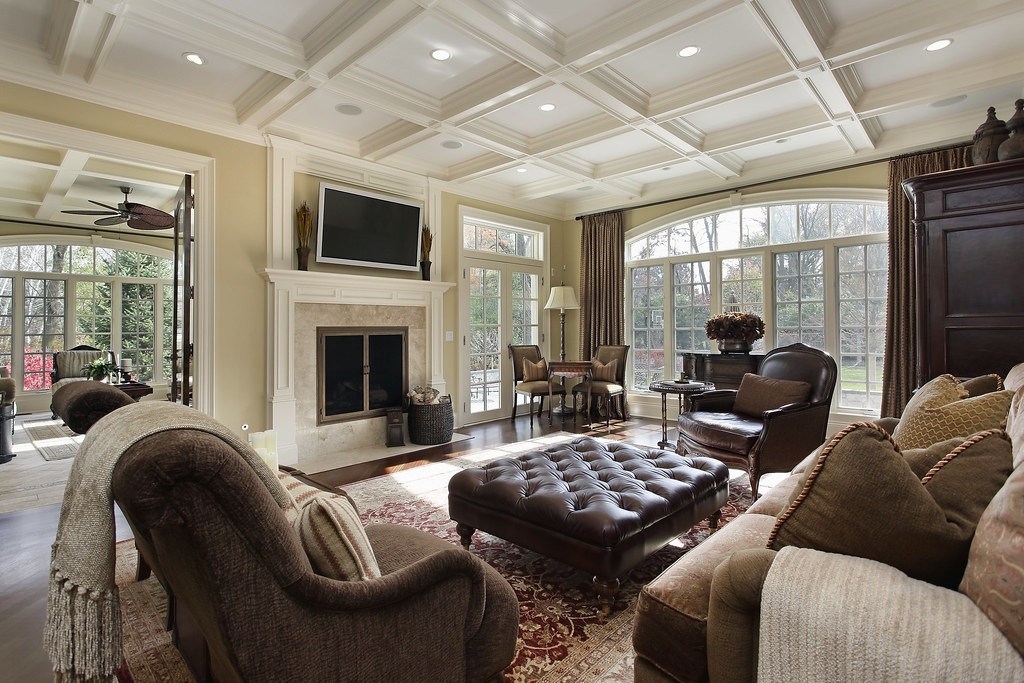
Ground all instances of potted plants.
[704,311,765,355]
[79,344,118,380]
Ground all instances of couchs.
[108,400,522,683]
[631,361,1024,683]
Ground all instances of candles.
[107,353,113,366]
[116,353,120,367]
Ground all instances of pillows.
[273,469,349,526]
[733,372,810,419]
[591,356,618,383]
[766,373,1014,590]
[291,496,381,584]
[522,356,548,383]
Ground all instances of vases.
[294,247,311,271]
[418,261,433,281]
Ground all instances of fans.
[60,185,176,231]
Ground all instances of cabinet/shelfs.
[901,155,1024,397]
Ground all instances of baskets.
[408,393,454,445]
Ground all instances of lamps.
[544,281,582,414]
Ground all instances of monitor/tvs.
[315,182,425,271]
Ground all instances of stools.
[449,435,731,614]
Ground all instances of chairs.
[507,343,630,428]
[51,379,361,633]
[48,344,118,426]
[675,342,838,500]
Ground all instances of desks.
[681,352,767,413]
[110,382,154,400]
[547,359,594,427]
[650,378,718,450]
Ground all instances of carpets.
[119,431,762,683]
[22,414,86,462]
